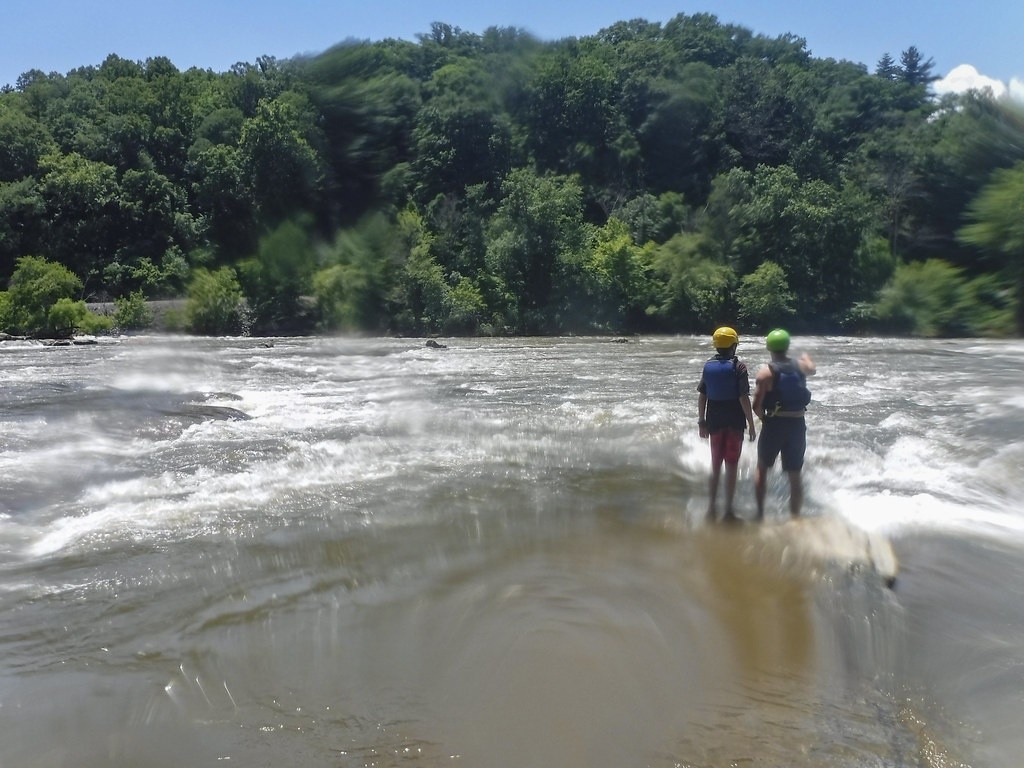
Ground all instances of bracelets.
[698,419,706,426]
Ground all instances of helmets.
[765,328,790,352]
[712,326,739,348]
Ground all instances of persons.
[696,327,757,527]
[752,328,818,523]
